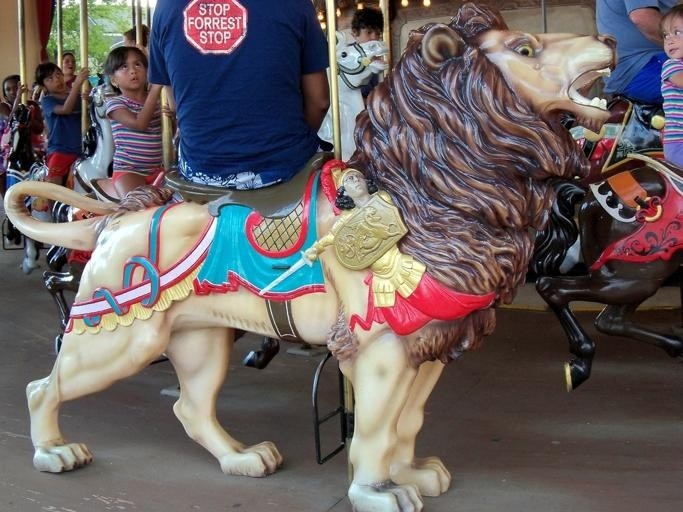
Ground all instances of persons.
[0,22,178,247]
[146,0,331,191]
[346,6,384,105]
[594,0,681,105]
[304,167,497,335]
[659,4,683,171]
